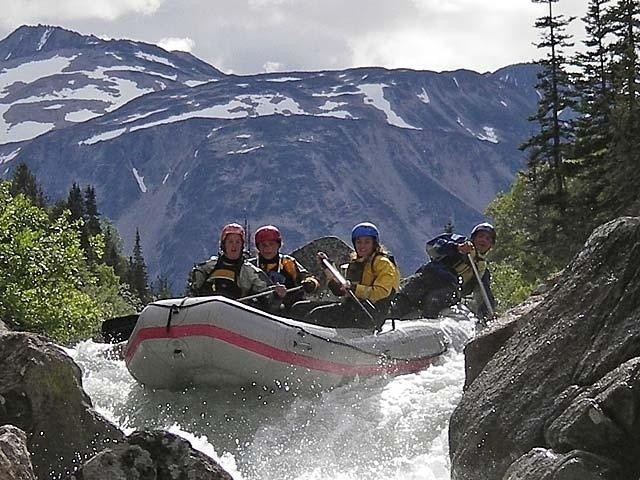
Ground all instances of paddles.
[321,255,375,324]
[101,289,278,344]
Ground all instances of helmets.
[221,223,245,241]
[255,225,281,243]
[469,222,496,245]
[352,222,379,243]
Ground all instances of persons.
[389,223,496,319]
[247,225,320,319]
[290,222,401,330]
[186,222,287,315]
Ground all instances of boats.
[121,293,474,392]
